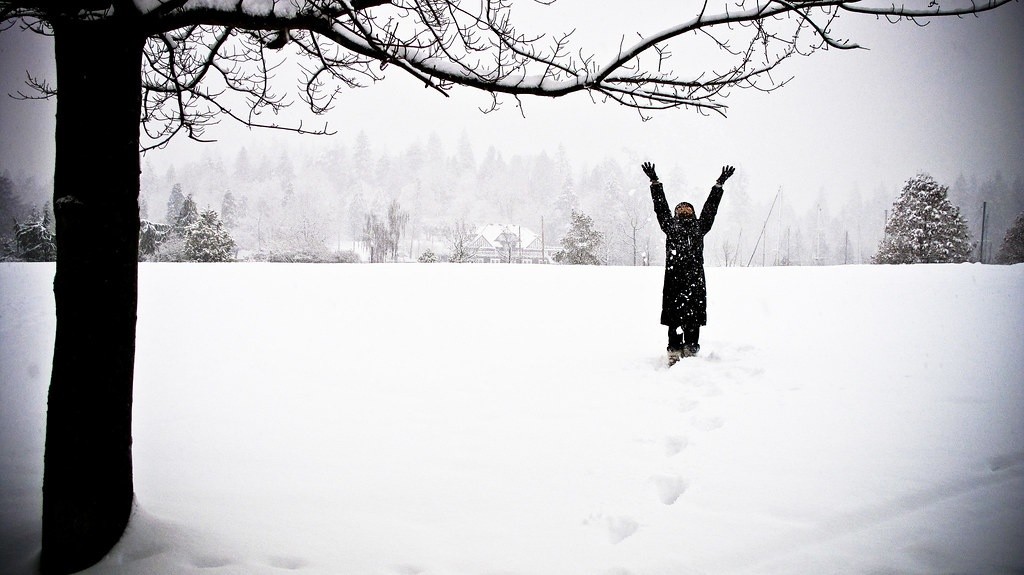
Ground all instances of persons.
[642,162,734,366]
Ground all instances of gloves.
[642,161,659,182]
[716,165,736,184]
